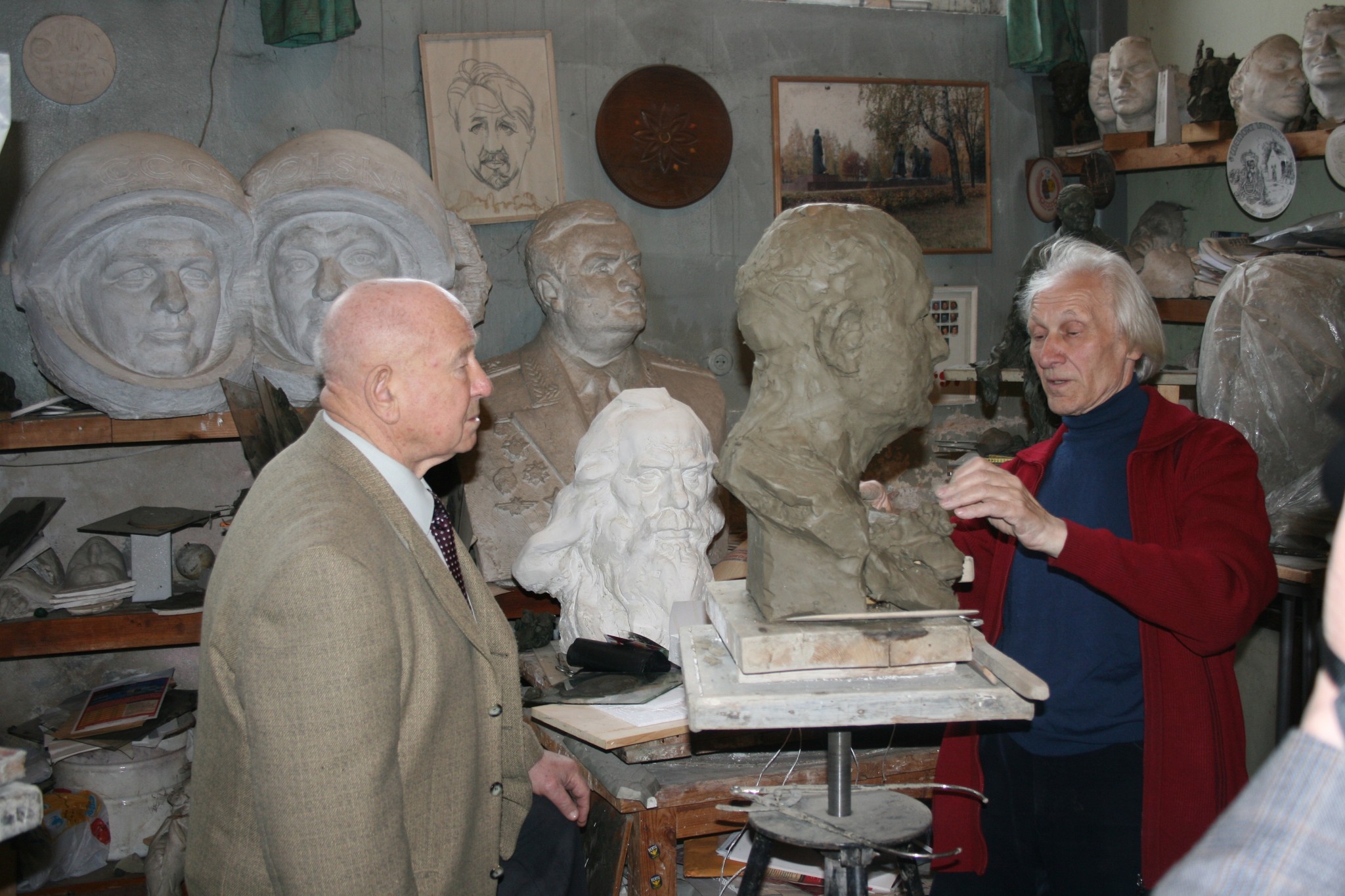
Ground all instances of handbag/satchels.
[565,637,672,683]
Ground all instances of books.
[1191,230,1345,298]
[716,828,905,895]
[932,438,1021,476]
[0,747,44,842]
[72,666,177,734]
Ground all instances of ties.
[427,492,471,611]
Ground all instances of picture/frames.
[771,75,993,254]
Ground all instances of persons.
[180,279,594,896]
[15,129,491,418]
[852,241,1282,896]
[1299,4,1345,129]
[710,201,966,618]
[1229,33,1310,135]
[1156,501,1344,896]
[514,386,732,658]
[452,198,729,577]
[0,537,65,619]
[989,184,1130,454]
[1186,40,1242,123]
[1131,200,1199,298]
[1088,34,1160,133]
[65,536,128,615]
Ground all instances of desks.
[543,736,942,895]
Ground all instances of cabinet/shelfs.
[0,407,320,660]
[1030,129,1335,327]
